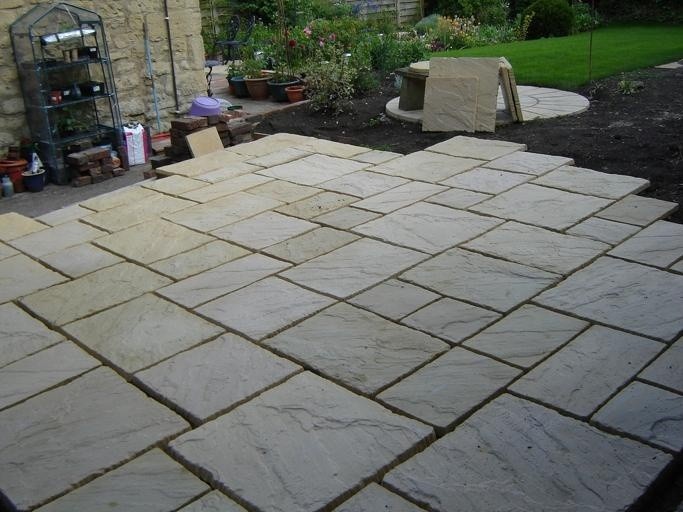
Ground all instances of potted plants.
[226,19,272,100]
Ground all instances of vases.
[1,158,45,192]
[267,75,306,103]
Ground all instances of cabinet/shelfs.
[8,4,130,185]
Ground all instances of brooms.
[143,23,171,142]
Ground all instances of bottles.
[2,177,13,196]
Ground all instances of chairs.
[201,15,255,97]
